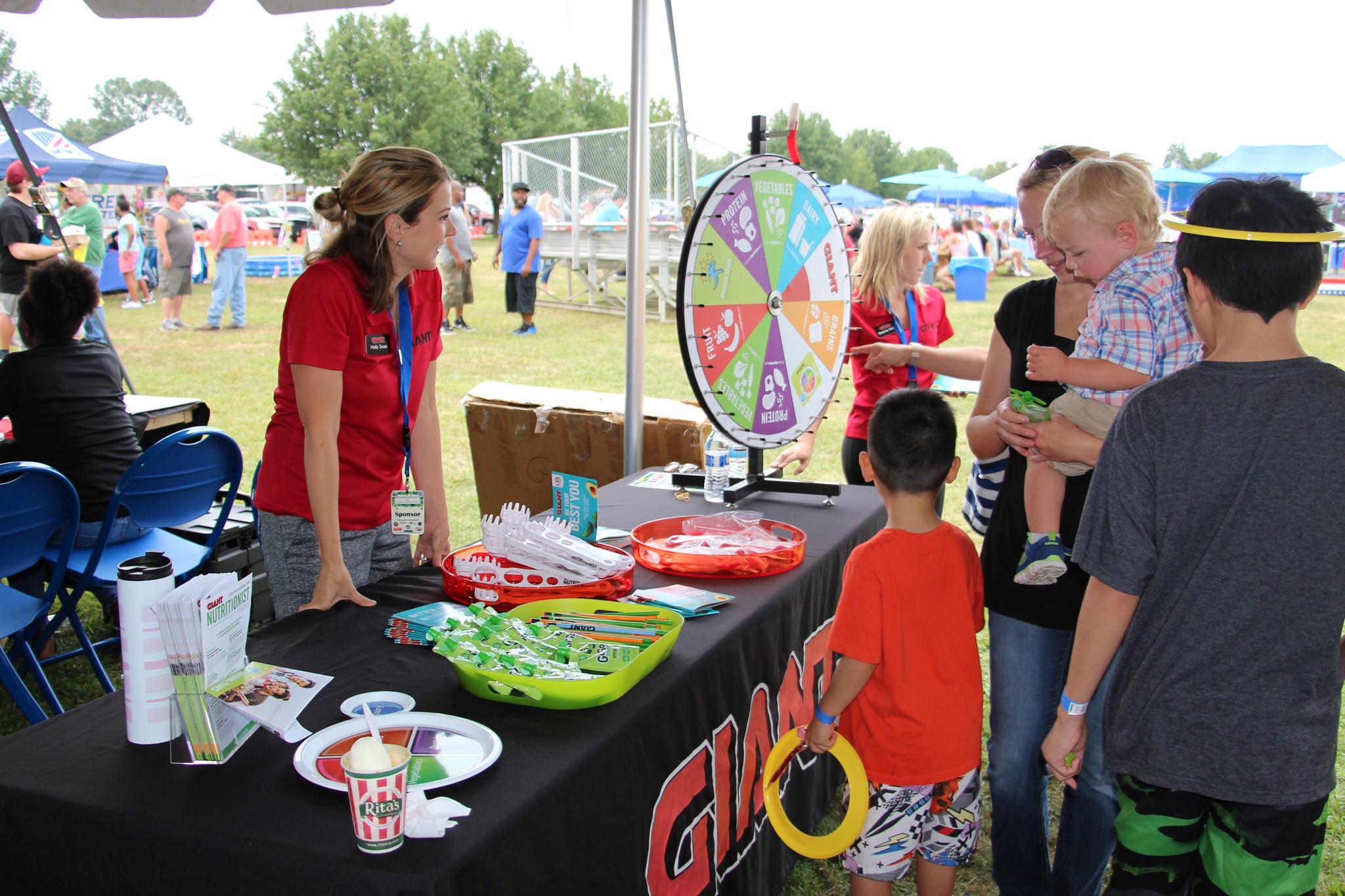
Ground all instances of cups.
[339,743,411,854]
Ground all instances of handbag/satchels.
[968,244,978,257]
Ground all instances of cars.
[140,198,317,243]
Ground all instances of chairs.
[0,424,243,723]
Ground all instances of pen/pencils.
[524,607,672,654]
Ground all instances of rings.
[420,556,429,561]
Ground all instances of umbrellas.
[1150,162,1221,216]
[695,144,1018,235]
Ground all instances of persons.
[966,145,1128,895]
[1042,175,1345,895]
[533,189,1042,303]
[0,158,106,364]
[1014,159,1208,586]
[220,666,316,706]
[809,388,985,896]
[252,148,458,618]
[0,259,159,664]
[439,180,478,335]
[770,206,954,519]
[103,185,249,333]
[492,181,544,333]
[850,341,990,382]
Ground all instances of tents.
[0,106,170,189]
[985,154,1037,227]
[1300,161,1345,202]
[1195,143,1345,183]
[87,114,305,189]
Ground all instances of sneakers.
[1014,531,1068,585]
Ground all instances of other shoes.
[159,319,179,333]
[452,316,473,332]
[194,324,220,331]
[225,324,244,330]
[121,300,142,309]
[439,319,453,333]
[509,322,537,337]
[173,319,189,327]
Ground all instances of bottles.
[117,549,183,746]
[704,424,730,503]
[728,441,747,479]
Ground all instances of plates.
[293,712,503,793]
[339,691,416,719]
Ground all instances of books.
[380,599,472,649]
[618,581,735,620]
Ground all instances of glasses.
[1025,148,1079,173]
[39,175,43,182]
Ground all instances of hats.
[511,182,530,192]
[6,160,50,185]
[212,184,235,194]
[165,187,189,198]
[59,177,87,192]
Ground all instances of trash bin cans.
[950,257,989,300]
[919,250,936,286]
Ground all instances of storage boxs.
[169,655,261,765]
[164,489,272,627]
[464,381,715,522]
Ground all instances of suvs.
[462,203,502,236]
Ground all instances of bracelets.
[908,341,921,367]
[815,703,841,727]
[1061,690,1089,716]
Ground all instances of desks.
[0,398,209,469]
[0,466,896,896]
